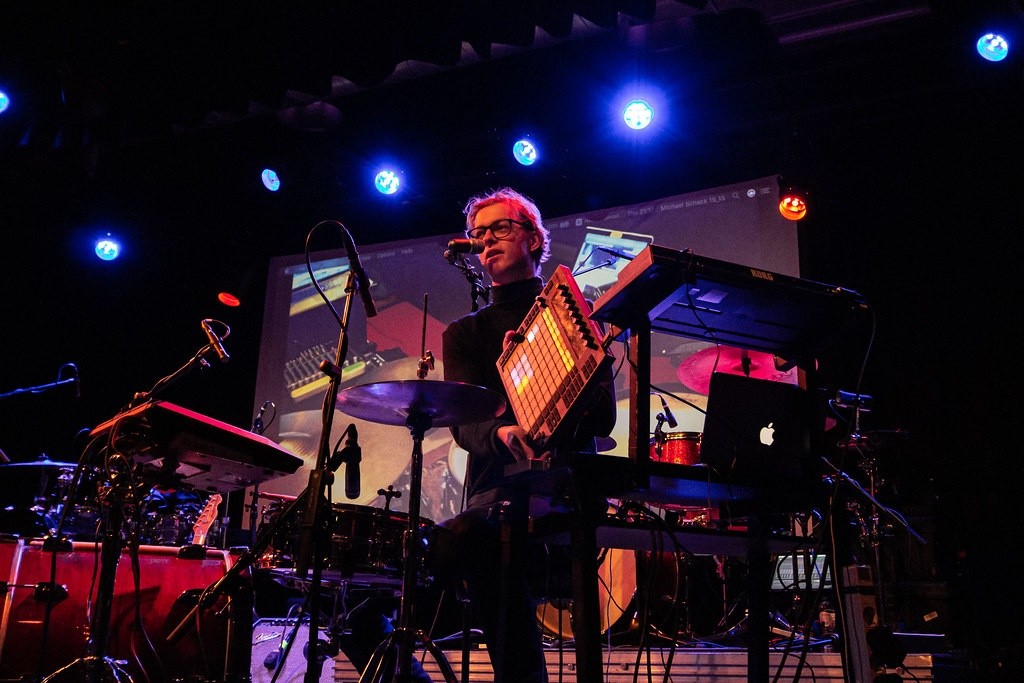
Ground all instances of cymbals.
[596,436,617,452]
[1,461,78,468]
[677,344,798,397]
[335,379,507,428]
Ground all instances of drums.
[646,432,716,512]
[535,499,681,644]
[0,543,252,683]
[258,501,436,594]
[20,460,213,548]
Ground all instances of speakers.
[643,554,749,649]
[250,619,336,683]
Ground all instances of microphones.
[448,238,486,254]
[75,369,80,398]
[201,321,230,362]
[263,629,294,669]
[345,424,360,500]
[342,229,378,318]
[252,402,267,433]
[598,246,636,261]
[661,398,677,428]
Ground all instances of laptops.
[572,226,655,304]
[694,373,812,478]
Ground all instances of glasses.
[466,218,530,242]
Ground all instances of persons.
[335,186,616,683]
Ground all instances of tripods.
[360,414,460,683]
[42,454,141,683]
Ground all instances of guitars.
[192,494,222,544]
